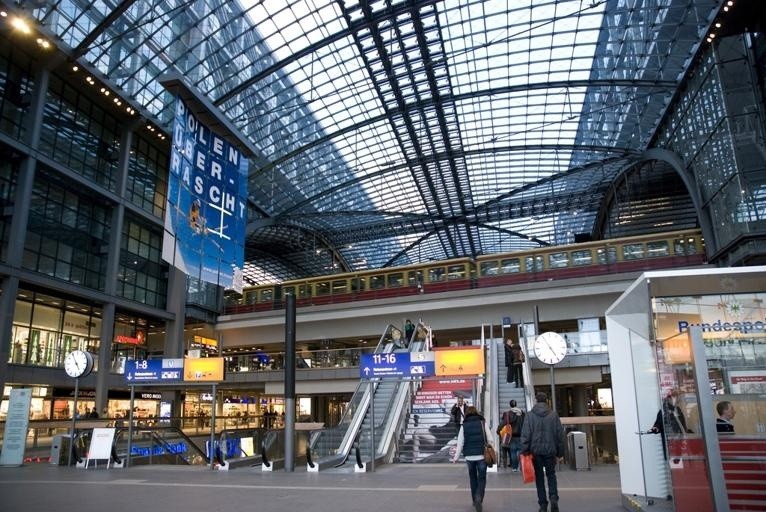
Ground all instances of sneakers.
[502,464,518,473]
[539,502,547,511]
[550,499,559,511]
[474,494,483,512]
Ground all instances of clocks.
[534,330,567,365]
[64,349,94,378]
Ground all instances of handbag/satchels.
[519,453,536,483]
[483,445,495,466]
[500,425,513,447]
[518,351,525,362]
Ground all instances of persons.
[651,393,687,460]
[452,405,495,512]
[521,394,565,512]
[404,320,438,348]
[190,199,208,237]
[63,404,158,440]
[503,336,524,388]
[715,401,737,433]
[496,400,525,472]
[195,408,212,431]
[593,399,603,416]
[228,406,285,429]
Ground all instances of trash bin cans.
[50,435,71,465]
[568,431,589,471]
